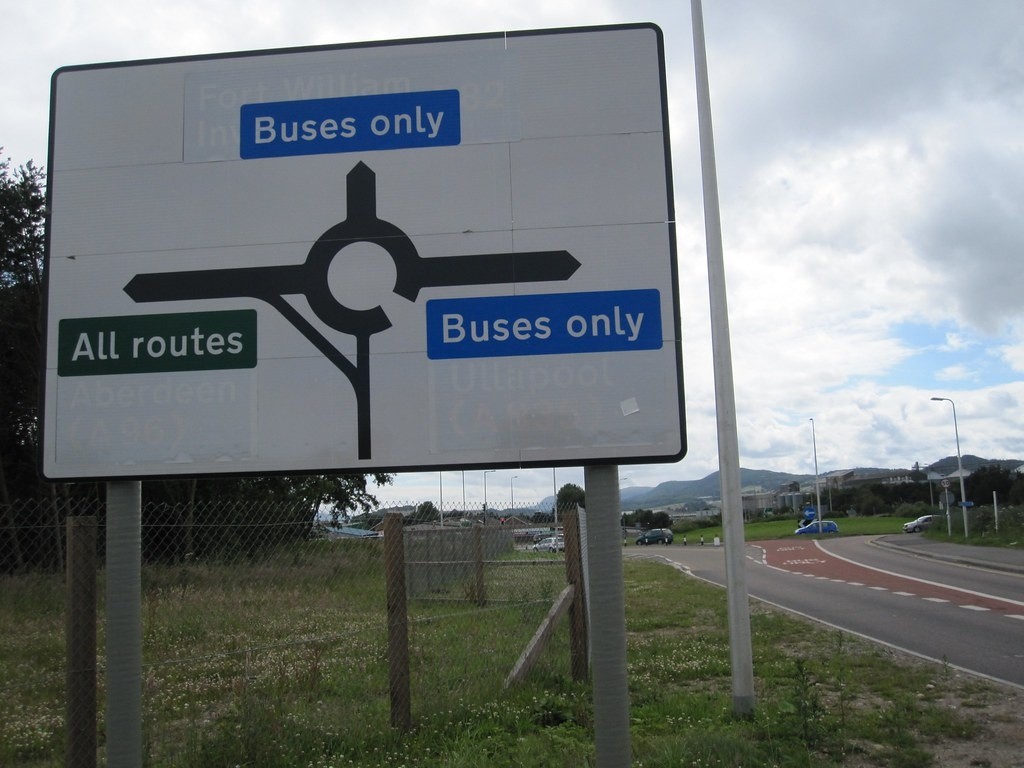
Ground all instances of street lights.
[931,398,970,539]
[809,418,823,534]
[511,476,518,532]
[484,470,496,525]
[619,477,628,481]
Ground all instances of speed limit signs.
[940,478,951,488]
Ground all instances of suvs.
[532,537,565,553]
[636,528,674,545]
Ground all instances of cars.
[533,534,553,543]
[794,521,839,534]
[903,514,942,533]
